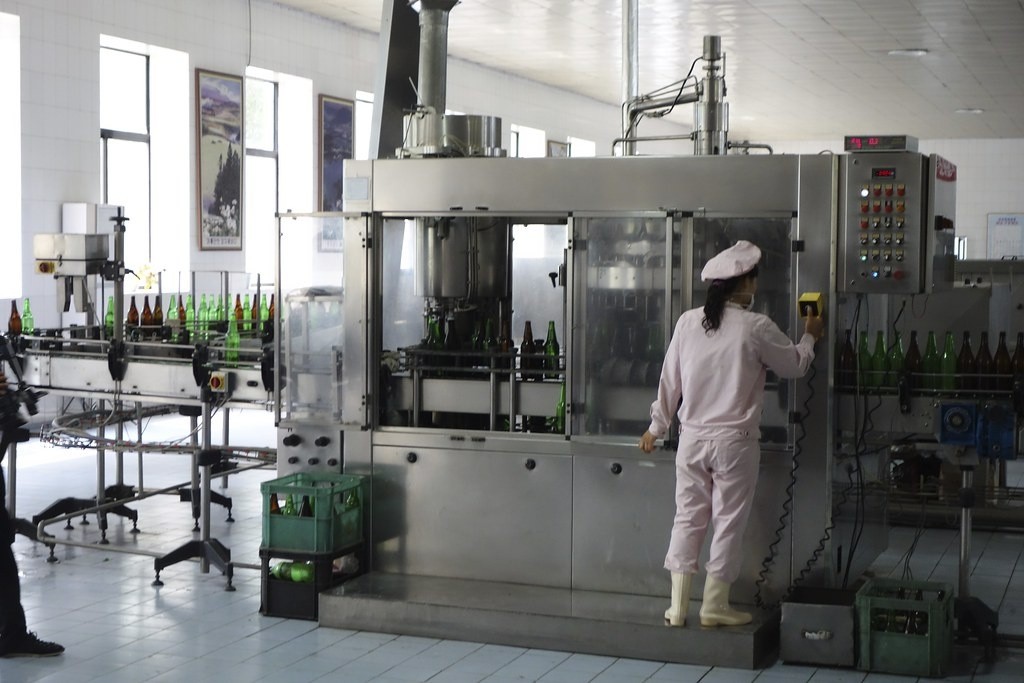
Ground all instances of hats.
[700,240,762,281]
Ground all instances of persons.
[638,242,821,628]
[0,373,66,657]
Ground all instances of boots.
[664,571,692,626]
[700,572,753,626]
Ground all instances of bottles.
[856,329,870,392]
[841,329,857,392]
[555,377,565,434]
[974,331,994,398]
[427,314,559,380]
[870,330,889,393]
[870,586,946,635]
[956,331,974,397]
[9,293,275,368]
[993,332,1012,397]
[270,478,361,582]
[904,331,922,396]
[1012,332,1024,398]
[923,331,941,397]
[887,330,905,394]
[940,332,958,397]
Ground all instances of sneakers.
[4,631,66,658]
